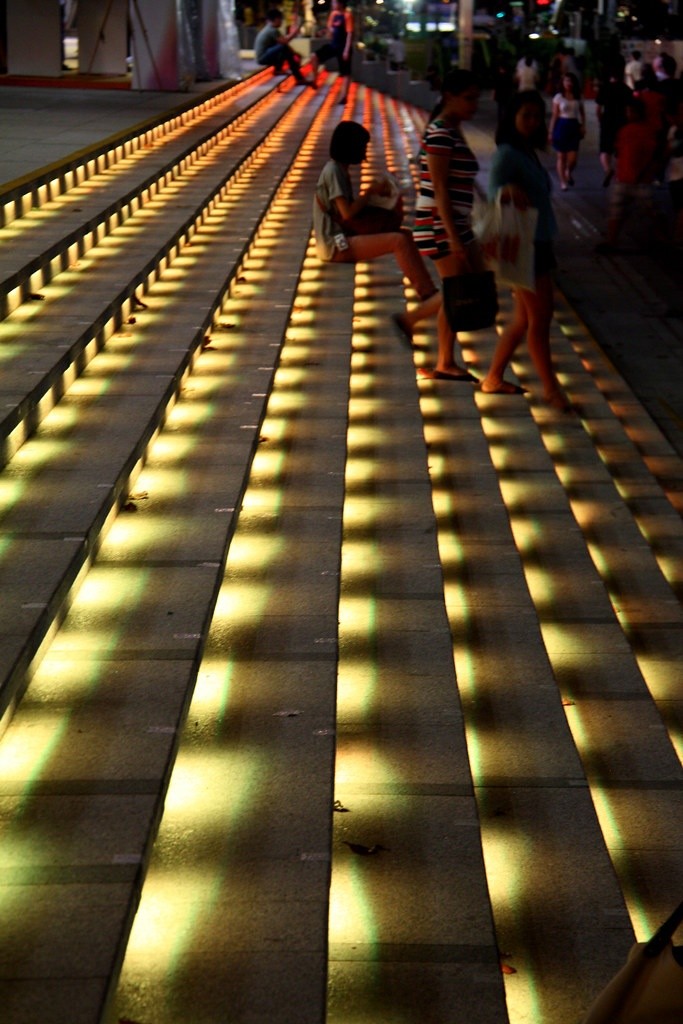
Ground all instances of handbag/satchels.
[346,211,387,235]
[441,269,499,333]
[470,185,539,292]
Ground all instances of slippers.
[489,384,525,395]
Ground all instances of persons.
[486,34,590,86]
[595,49,682,274]
[391,69,481,383]
[548,72,587,191]
[305,0,354,104]
[480,87,583,418]
[388,34,408,71]
[254,9,313,85]
[312,120,438,301]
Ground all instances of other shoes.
[432,371,480,383]
[389,313,413,355]
